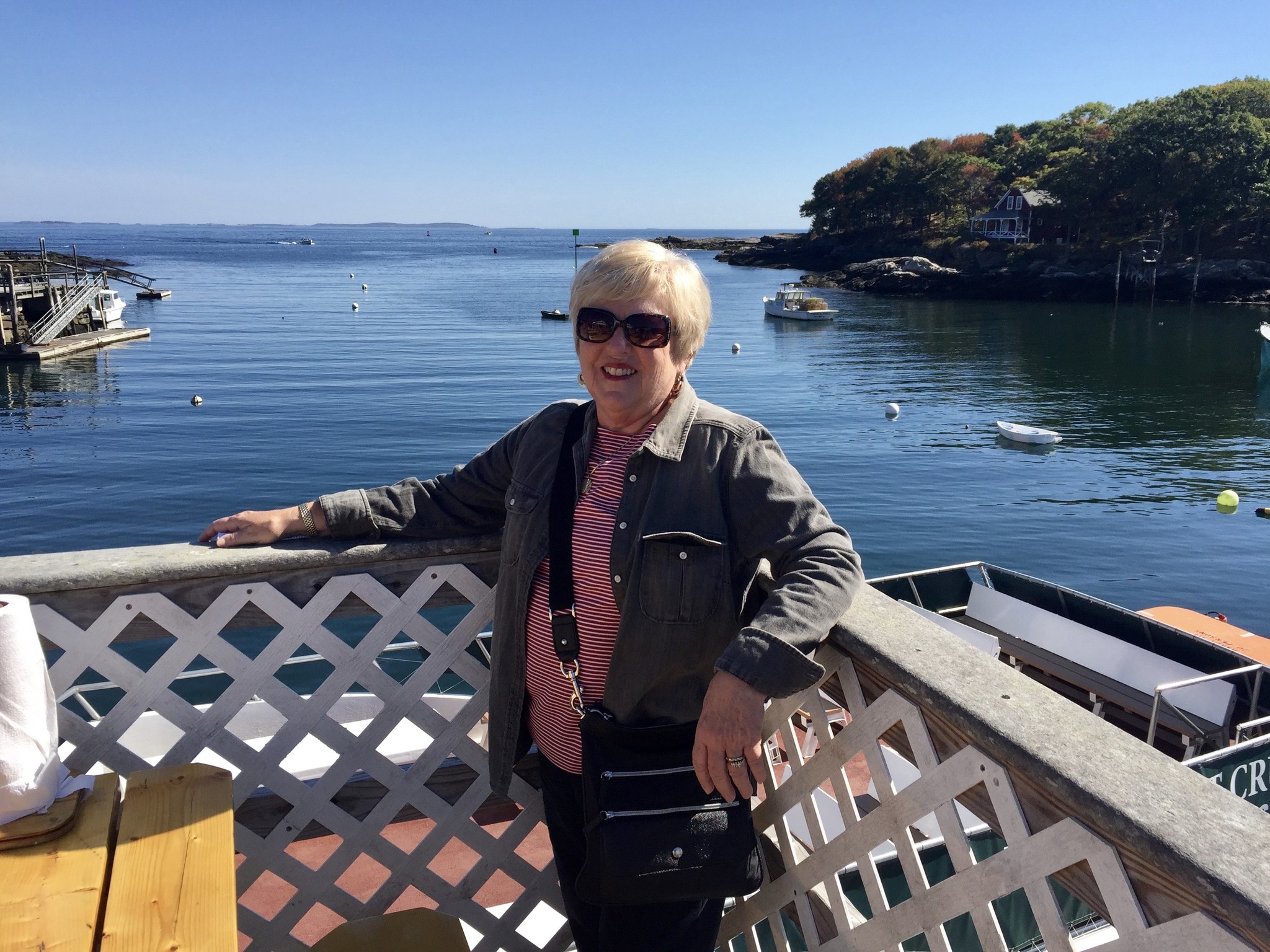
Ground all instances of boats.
[82,289,127,324]
[996,420,1059,445]
[762,282,839,321]
[298,237,313,245]
[540,310,570,319]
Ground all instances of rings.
[726,755,746,768]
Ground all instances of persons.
[198,240,865,952]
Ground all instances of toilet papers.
[0,594,96,826]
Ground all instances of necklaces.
[581,395,672,494]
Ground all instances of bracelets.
[298,502,319,536]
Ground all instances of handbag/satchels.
[583,705,764,907]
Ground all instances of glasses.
[576,307,680,348]
